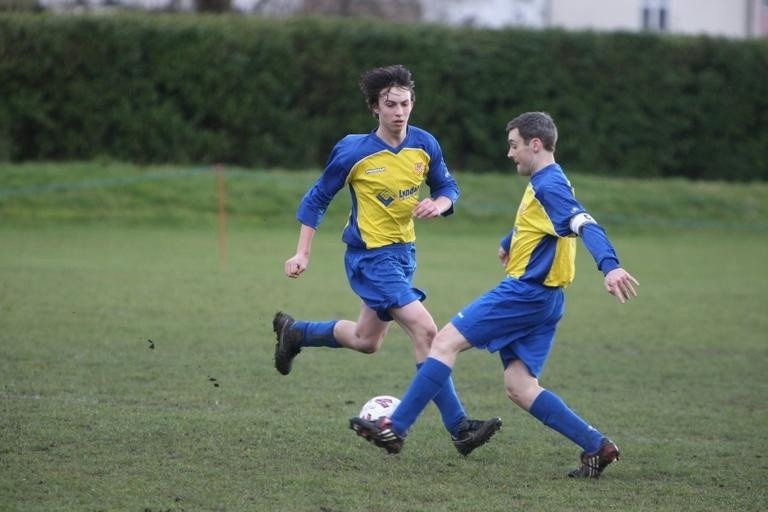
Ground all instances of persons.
[273,65,502,455]
[352,112,641,479]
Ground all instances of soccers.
[359,396,402,423]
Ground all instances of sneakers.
[272,311,301,376]
[452,416,502,457]
[568,440,621,480]
[348,415,403,455]
